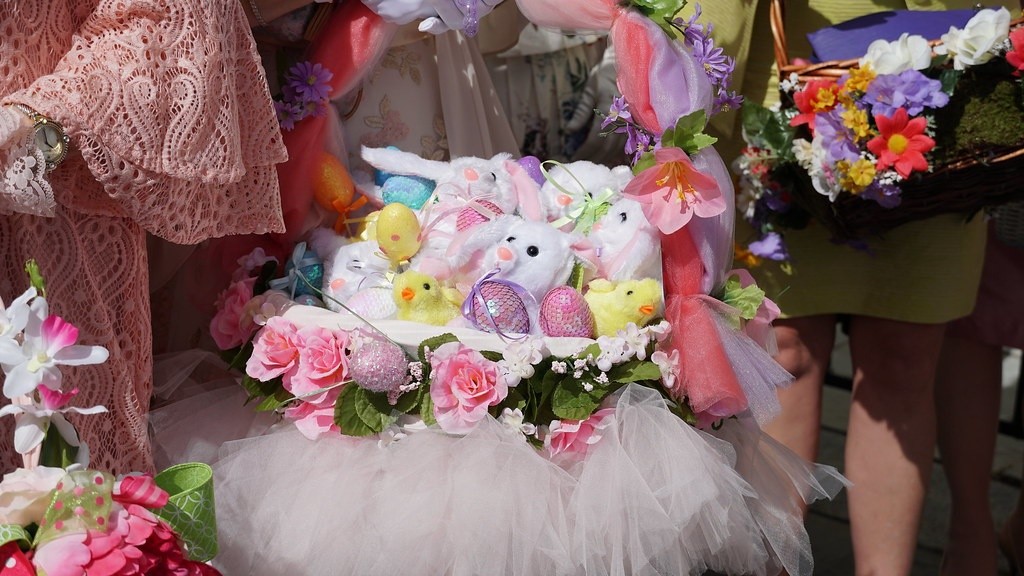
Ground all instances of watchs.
[13,103,68,175]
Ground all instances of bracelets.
[250,0,265,26]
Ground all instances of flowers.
[0,259,205,576]
[208,0,1024,459]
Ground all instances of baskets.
[770,1,1024,240]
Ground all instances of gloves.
[361,0,504,35]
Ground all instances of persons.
[241,0,1024,576]
[0,0,289,470]
